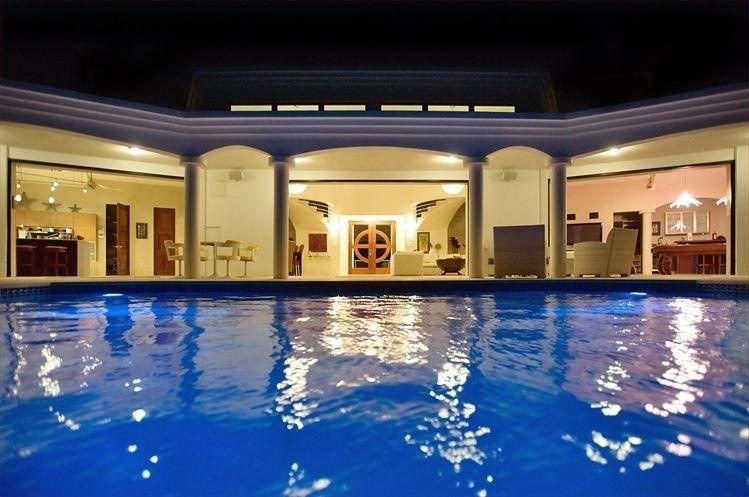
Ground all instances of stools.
[44,246,70,277]
[17,245,37,277]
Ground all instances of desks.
[651,239,726,275]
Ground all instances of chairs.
[439,253,461,275]
[164,240,259,278]
[423,253,439,275]
[573,228,639,279]
[461,255,467,275]
[390,250,422,276]
[493,224,551,279]
[293,244,304,276]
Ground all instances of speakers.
[502,172,517,183]
[228,171,243,180]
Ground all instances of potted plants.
[436,254,466,276]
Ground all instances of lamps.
[289,183,307,192]
[668,169,702,209]
[14,166,90,204]
[441,185,466,195]
[716,165,733,208]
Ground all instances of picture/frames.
[136,223,148,239]
